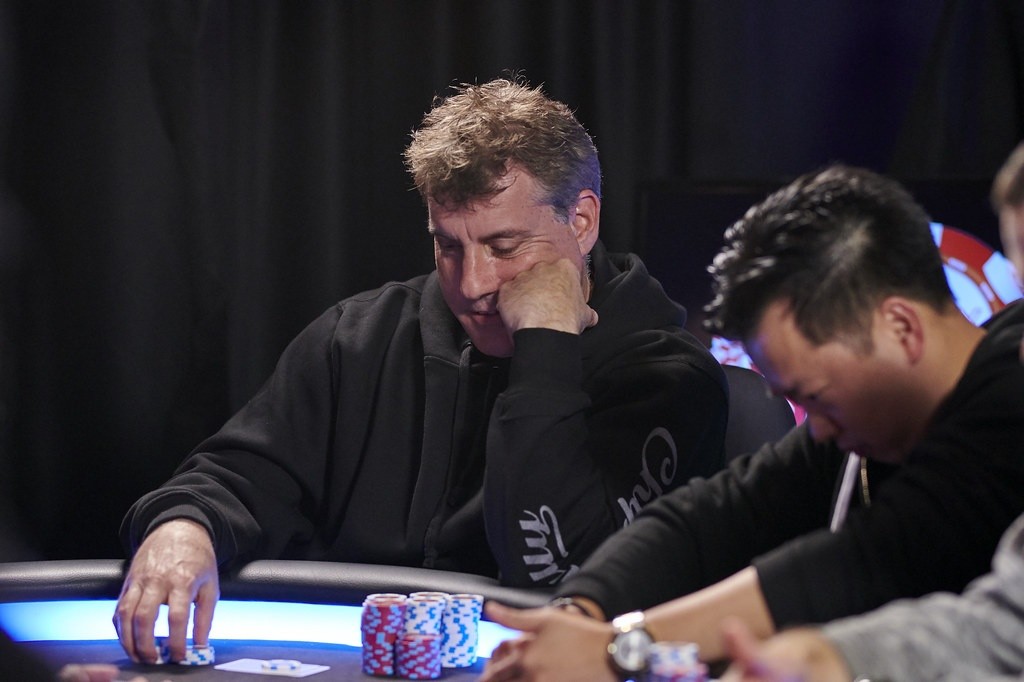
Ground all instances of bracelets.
[550,597,592,618]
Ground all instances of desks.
[0,561,547,682]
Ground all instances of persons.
[112,79,729,663]
[479,168,1024,682]
[718,514,1024,682]
[996,149,1024,287]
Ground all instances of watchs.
[607,611,655,682]
